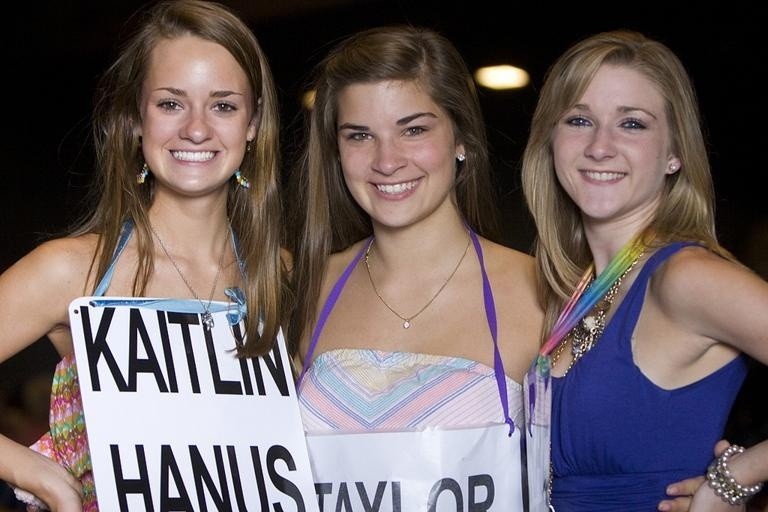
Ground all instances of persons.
[295,26,548,511]
[1,1,302,510]
[516,30,767,512]
[1,360,54,509]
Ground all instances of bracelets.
[705,442,765,507]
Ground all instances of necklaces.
[546,229,657,377]
[148,220,233,332]
[361,228,474,331]
